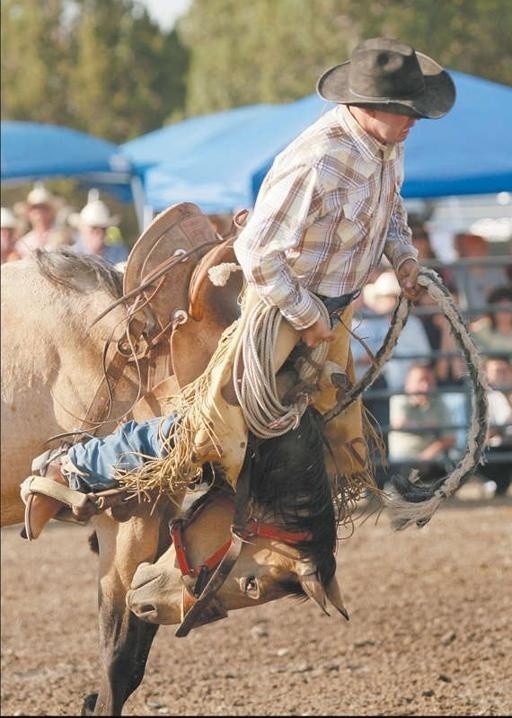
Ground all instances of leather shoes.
[25,445,69,541]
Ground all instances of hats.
[1,208,17,229]
[26,188,52,205]
[68,200,121,228]
[317,37,455,119]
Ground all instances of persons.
[22,36,458,543]
[344,213,511,515]
[1,185,131,276]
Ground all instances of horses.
[0,241,350,717]
